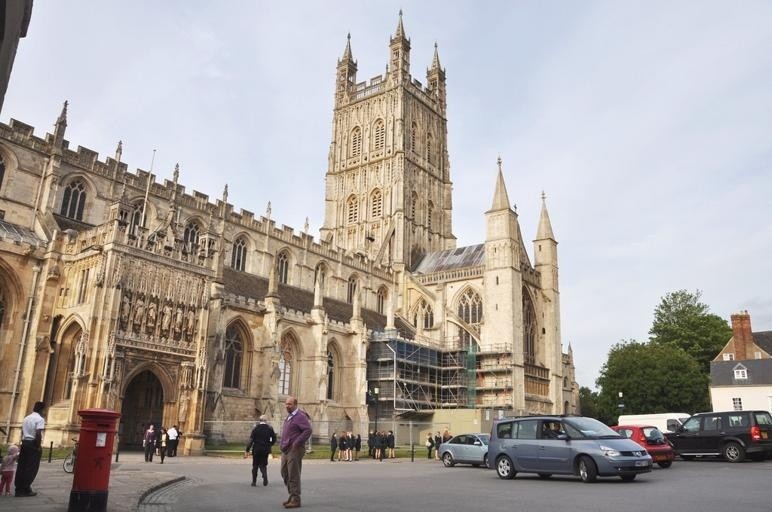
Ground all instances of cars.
[438,433,492,468]
[612,425,674,469]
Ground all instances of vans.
[488,414,653,482]
[618,411,772,462]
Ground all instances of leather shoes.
[15,491,20,497]
[19,492,36,497]
[283,499,290,505]
[285,501,300,508]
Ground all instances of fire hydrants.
[65,409,121,509]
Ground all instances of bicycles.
[65,439,78,472]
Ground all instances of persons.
[426,429,455,460]
[245,414,274,486]
[552,423,563,438]
[329,425,401,462]
[15,400,47,496]
[143,421,183,464]
[122,291,197,341]
[278,396,314,508]
[1,444,21,496]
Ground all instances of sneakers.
[5,493,11,497]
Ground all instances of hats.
[7,445,18,455]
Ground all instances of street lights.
[367,385,382,458]
